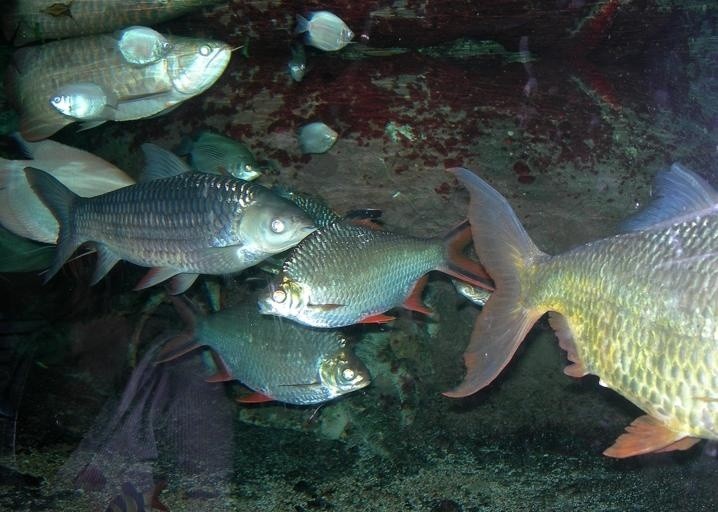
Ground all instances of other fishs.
[1,0,356,297]
